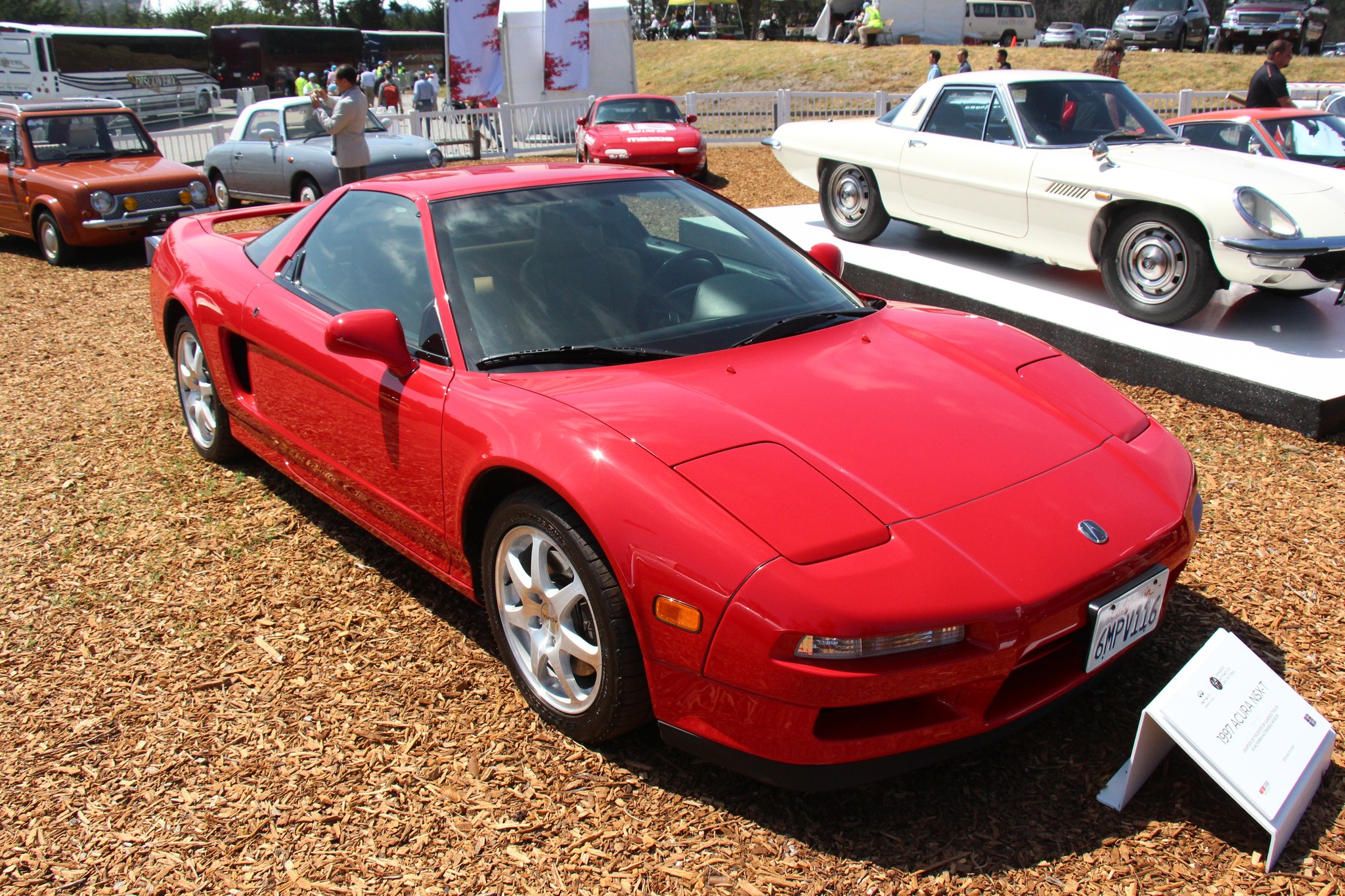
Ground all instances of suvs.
[1043,22,1094,50]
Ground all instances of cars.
[1319,40,1345,57]
[203,97,445,211]
[1112,0,1211,52]
[1085,29,1113,49]
[1127,91,1345,171]
[0,97,220,267]
[1205,25,1221,54]
[574,94,708,180]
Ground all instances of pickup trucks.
[1219,0,1329,57]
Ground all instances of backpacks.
[382,82,398,106]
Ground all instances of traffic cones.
[1011,36,1016,47]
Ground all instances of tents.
[664,0,745,41]
[811,0,966,46]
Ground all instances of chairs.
[933,103,991,141]
[256,121,280,133]
[68,124,97,146]
[600,109,619,121]
[520,204,647,343]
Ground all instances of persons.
[646,2,884,49]
[926,49,942,82]
[1232,40,1319,161]
[275,60,503,149]
[1000,101,1055,145]
[311,63,371,187]
[996,49,1012,70]
[957,48,972,73]
[1092,38,1126,131]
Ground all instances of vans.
[963,0,1036,48]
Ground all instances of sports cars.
[143,162,1204,764]
[759,69,1345,326]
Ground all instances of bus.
[0,21,221,138]
[208,24,446,99]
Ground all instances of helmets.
[428,64,434,70]
[299,72,306,77]
[308,73,316,78]
[331,65,338,71]
[324,70,328,73]
[328,83,338,91]
[398,62,403,66]
[378,61,383,65]
[863,2,870,9]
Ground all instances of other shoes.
[487,139,490,148]
[860,43,869,49]
[499,144,502,148]
